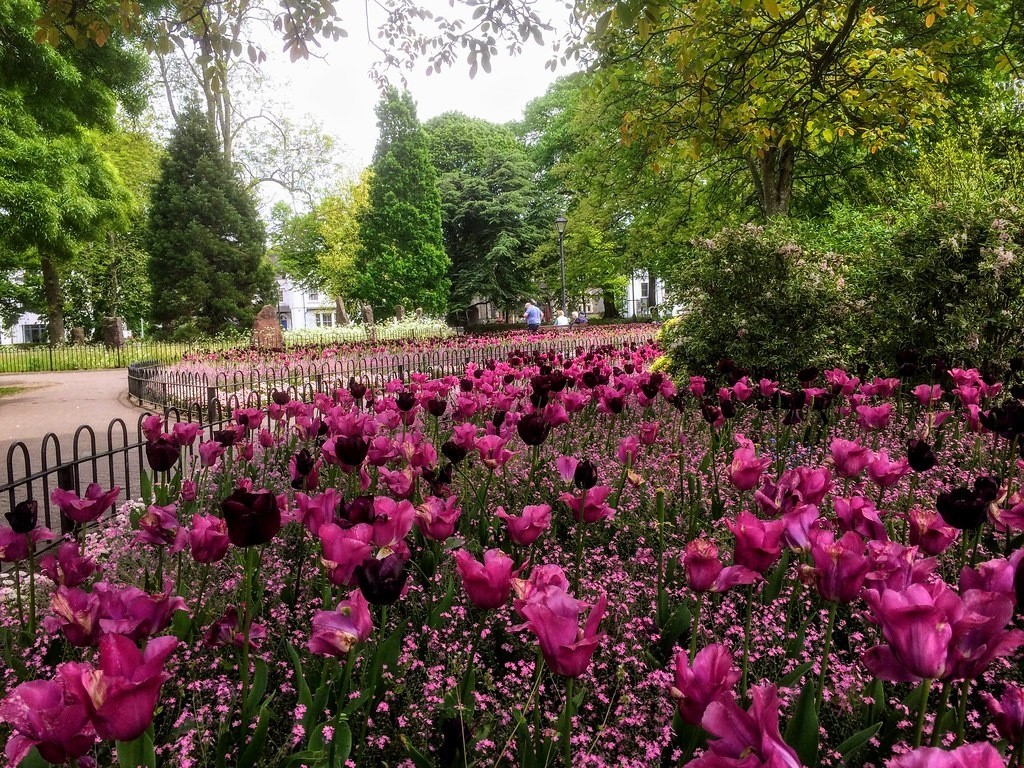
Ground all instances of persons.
[553,310,588,330]
[523,302,544,332]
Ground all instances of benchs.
[537,324,587,330]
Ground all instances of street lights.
[555,216,569,316]
